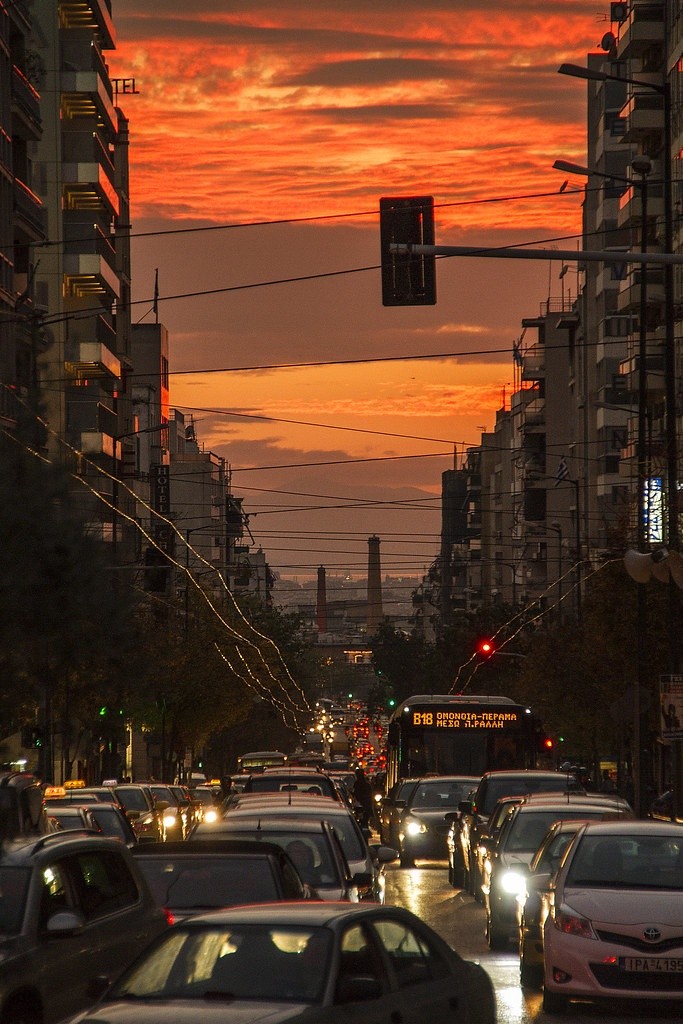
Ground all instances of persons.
[582,770,614,793]
[215,777,237,807]
[662,694,680,728]
[354,769,372,838]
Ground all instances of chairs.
[594,840,622,881]
[523,821,547,845]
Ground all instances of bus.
[383,694,547,801]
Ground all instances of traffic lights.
[32,734,46,749]
[558,733,565,745]
[97,701,113,739]
[544,732,555,751]
[478,638,491,664]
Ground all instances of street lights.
[479,557,517,610]
[553,159,649,823]
[526,471,583,626]
[558,62,681,821]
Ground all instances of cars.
[56,901,497,1023]
[511,819,681,992]
[1,692,646,955]
[530,823,682,1014]
[124,839,315,926]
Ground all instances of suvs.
[1,825,176,1024]
